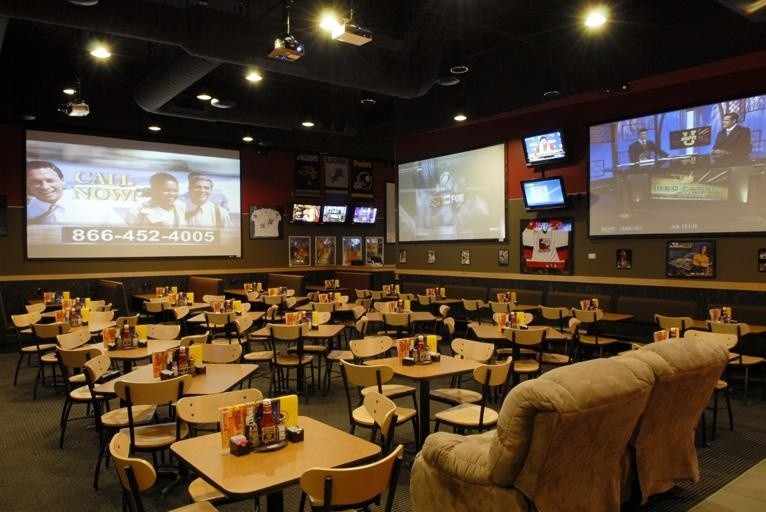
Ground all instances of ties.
[185,207,202,220]
[27,204,57,224]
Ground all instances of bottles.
[172,362,178,377]
[260,399,276,446]
[65,297,90,326]
[408,336,432,362]
[117,336,123,347]
[277,419,287,441]
[189,358,195,373]
[249,422,260,447]
[179,346,190,377]
[124,324,131,347]
[132,334,138,346]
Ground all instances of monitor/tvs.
[520,175,569,213]
[520,126,574,168]
[349,205,379,227]
[289,201,322,226]
[320,203,350,227]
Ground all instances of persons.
[125,171,184,226]
[629,128,674,162]
[711,113,751,159]
[179,171,230,212]
[692,244,709,273]
[534,137,554,156]
[184,176,233,228]
[26,161,87,224]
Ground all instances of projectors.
[58,100,91,118]
[264,34,307,65]
[331,22,374,48]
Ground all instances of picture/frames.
[288,235,311,267]
[365,236,385,267]
[342,236,363,267]
[665,238,717,278]
[315,236,337,266]
[498,249,509,266]
[426,249,436,264]
[399,249,406,264]
[461,249,471,265]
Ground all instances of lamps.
[58,79,89,116]
[265,0,305,63]
